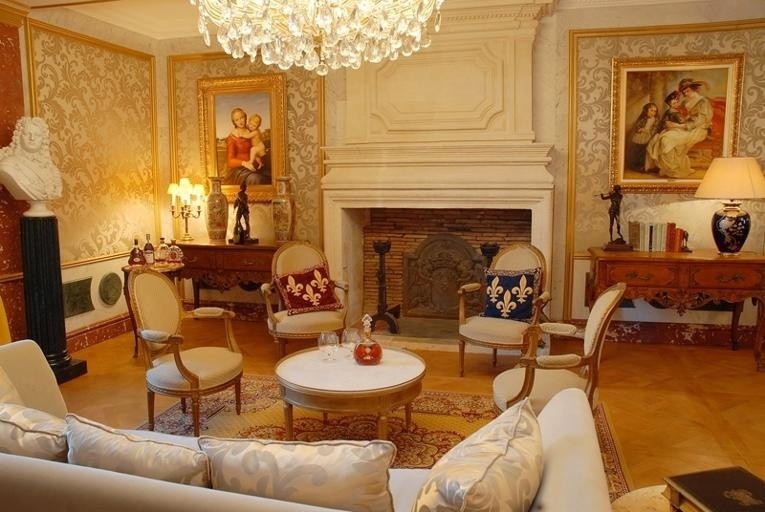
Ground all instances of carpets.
[129,373,632,505]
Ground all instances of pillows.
[64,412,212,490]
[411,397,545,512]
[198,433,397,511]
[1,401,71,463]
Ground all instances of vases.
[270,178,298,244]
[202,176,230,247]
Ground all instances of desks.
[586,242,765,378]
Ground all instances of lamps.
[165,178,206,243]
[187,0,451,80]
[693,156,760,256]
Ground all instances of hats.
[680,79,702,91]
[664,88,676,102]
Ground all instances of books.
[661,467,765,512]
[629,220,685,252]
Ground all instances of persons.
[633,103,659,171]
[643,78,713,179]
[237,114,267,172]
[657,91,698,134]
[228,184,259,245]
[0,115,64,217]
[594,185,634,251]
[222,108,271,184]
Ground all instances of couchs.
[1,339,613,511]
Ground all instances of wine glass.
[315,330,339,365]
[340,328,362,361]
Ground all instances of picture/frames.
[195,70,291,206]
[609,49,747,199]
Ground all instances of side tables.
[123,260,185,357]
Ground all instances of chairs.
[260,240,351,362]
[490,279,630,421]
[457,244,550,377]
[124,268,245,436]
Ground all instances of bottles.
[128,239,146,268]
[166,239,184,267]
[153,236,171,270]
[141,234,155,266]
[353,313,383,366]
